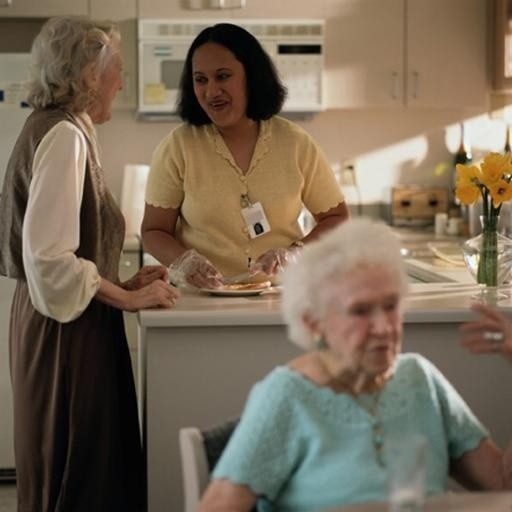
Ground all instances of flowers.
[454,148,512,209]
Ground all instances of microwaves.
[136,21,323,116]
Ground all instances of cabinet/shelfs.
[320,0,494,110]
[135,0,320,114]
[0,2,134,109]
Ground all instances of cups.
[435,214,465,235]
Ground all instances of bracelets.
[290,240,304,247]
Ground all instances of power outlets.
[341,160,356,184]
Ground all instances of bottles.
[503,124,511,151]
[453,120,472,203]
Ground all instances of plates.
[199,289,263,298]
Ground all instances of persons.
[0,16,181,512]
[196,216,512,512]
[141,23,351,290]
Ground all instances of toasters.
[392,188,447,227]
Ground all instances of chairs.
[177,417,238,511]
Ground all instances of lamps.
[120,164,150,238]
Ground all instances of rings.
[483,332,503,342]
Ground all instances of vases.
[476,206,499,285]
